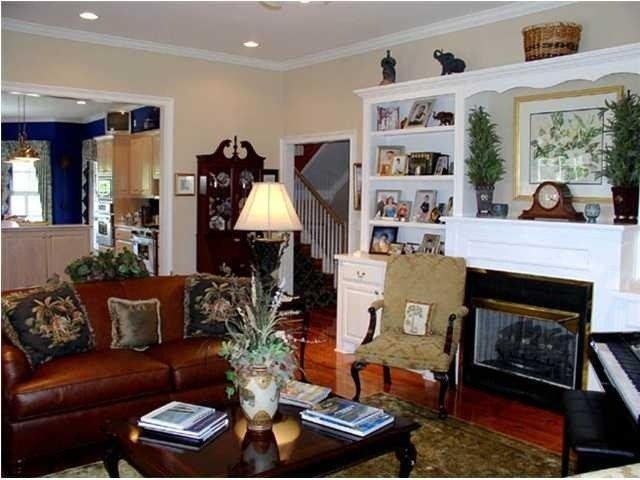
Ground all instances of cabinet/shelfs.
[196,136,266,275]
[332,255,388,354]
[114,134,160,199]
[458,43,640,225]
[1,225,93,290]
[360,74,459,260]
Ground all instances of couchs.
[1,276,239,478]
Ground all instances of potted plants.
[591,90,640,224]
[464,105,508,217]
[216,273,327,430]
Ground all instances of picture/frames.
[369,99,455,254]
[512,85,624,205]
[175,172,196,196]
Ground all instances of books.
[301,420,399,442]
[136,400,228,439]
[279,378,395,437]
[137,423,231,454]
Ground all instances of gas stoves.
[141,223,159,231]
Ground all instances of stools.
[561,390,640,478]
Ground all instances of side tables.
[261,288,311,383]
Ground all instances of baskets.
[523,22,582,61]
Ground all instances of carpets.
[37,389,576,478]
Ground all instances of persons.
[380,110,391,129]
[394,158,402,174]
[382,197,396,217]
[419,194,430,219]
[381,151,394,175]
[397,203,408,217]
[408,104,426,125]
[424,239,435,254]
[372,232,389,256]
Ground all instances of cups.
[487,203,508,218]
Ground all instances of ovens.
[131,228,158,274]
[93,173,114,247]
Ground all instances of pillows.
[398,296,437,336]
[107,296,162,349]
[183,273,262,340]
[1,280,97,369]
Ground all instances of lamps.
[233,182,304,297]
[2,95,41,163]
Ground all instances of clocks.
[518,182,586,221]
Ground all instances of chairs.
[350,254,470,420]
